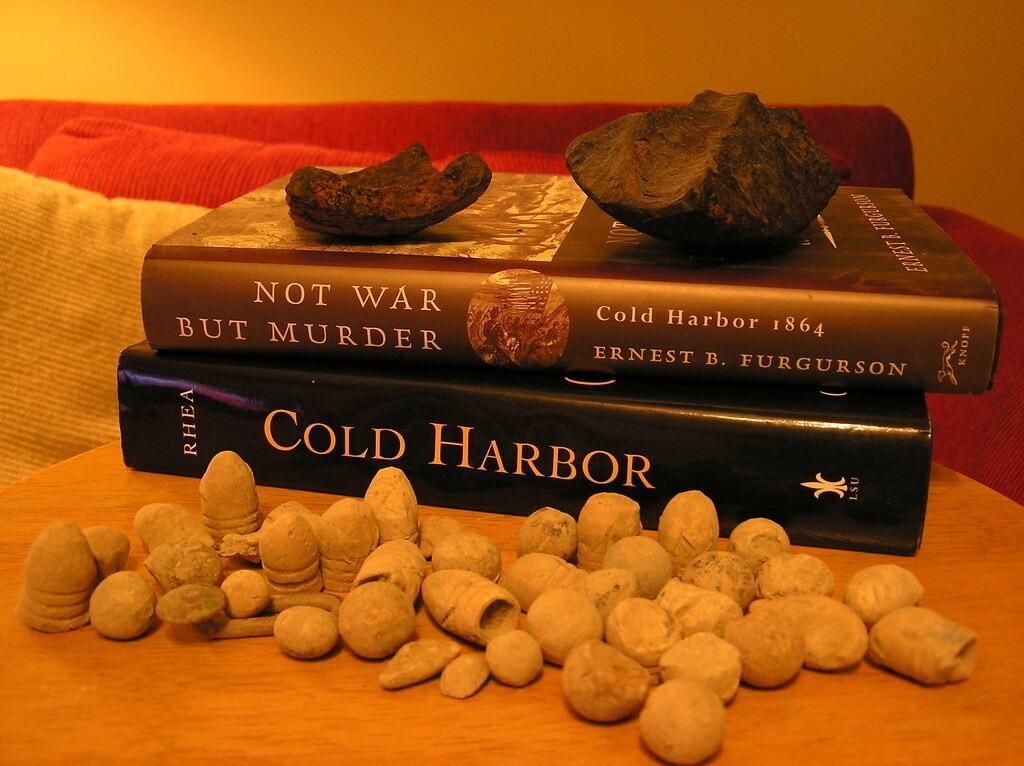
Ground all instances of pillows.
[31,119,440,321]
[0,162,216,492]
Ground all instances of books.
[117,342,935,556]
[141,166,1003,395]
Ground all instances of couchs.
[0,101,1024,505]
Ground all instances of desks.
[0,443,1024,766]
